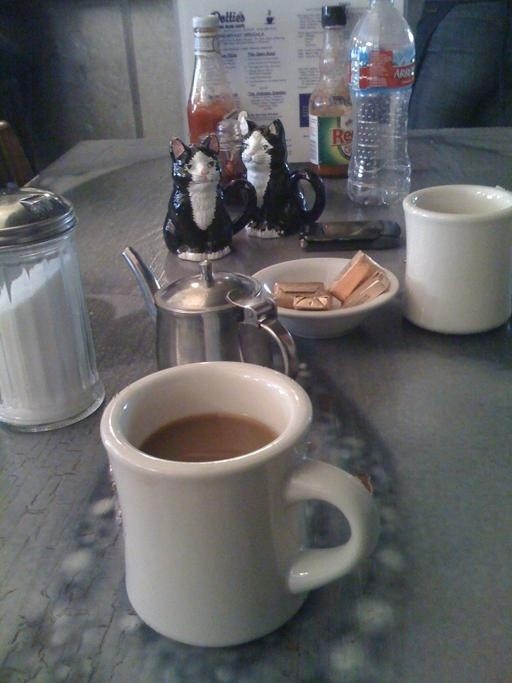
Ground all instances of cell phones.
[300,220,400,251]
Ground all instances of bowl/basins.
[247,255,401,341]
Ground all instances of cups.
[94,355,382,650]
[398,181,512,337]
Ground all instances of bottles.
[343,0,418,208]
[183,12,241,190]
[0,181,107,435]
[307,2,355,179]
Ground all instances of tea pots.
[118,245,299,380]
